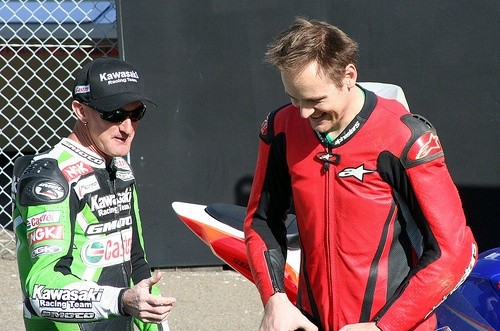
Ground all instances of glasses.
[83,103,148,123]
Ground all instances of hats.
[71,57,157,114]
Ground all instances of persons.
[12,55,176,331]
[243,16,479,330]
